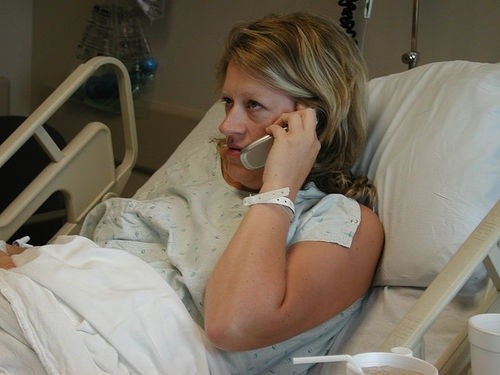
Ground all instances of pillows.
[352,60,499,288]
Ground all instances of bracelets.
[240,187,296,215]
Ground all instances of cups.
[466,313,500,375]
[345,351,439,375]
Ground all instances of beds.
[1,56,499,375]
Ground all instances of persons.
[2,10,385,375]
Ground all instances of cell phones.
[239,108,320,170]
